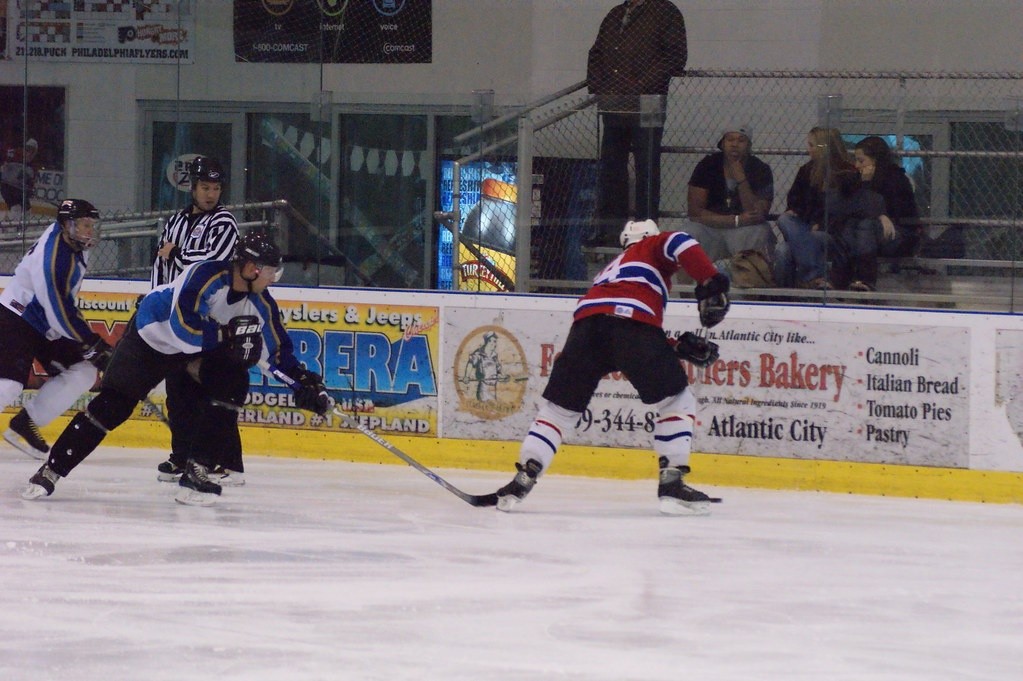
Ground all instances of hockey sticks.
[139,395,171,429]
[256,359,499,507]
[695,327,708,401]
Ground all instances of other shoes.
[810,278,832,290]
[849,281,869,291]
[584,232,617,246]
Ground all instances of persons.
[22,233,328,506]
[0,199,116,461]
[586,0,688,256]
[497,219,729,515]
[0,138,41,240]
[150,155,245,486]
[690,124,921,295]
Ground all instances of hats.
[717,123,752,150]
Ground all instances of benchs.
[530,248,1023,313]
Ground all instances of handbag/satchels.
[726,249,776,288]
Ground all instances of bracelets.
[737,178,747,185]
[735,216,739,228]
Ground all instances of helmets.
[57,199,100,250]
[234,229,282,267]
[189,156,225,183]
[619,219,660,248]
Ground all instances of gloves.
[288,370,329,414]
[675,332,719,367]
[77,332,114,370]
[228,315,263,366]
[695,273,729,329]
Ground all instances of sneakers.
[209,465,245,487]
[657,456,711,516]
[496,458,543,511]
[157,453,182,483]
[21,462,60,498]
[3,406,51,459]
[175,458,221,505]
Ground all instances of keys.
[726,198,731,208]
